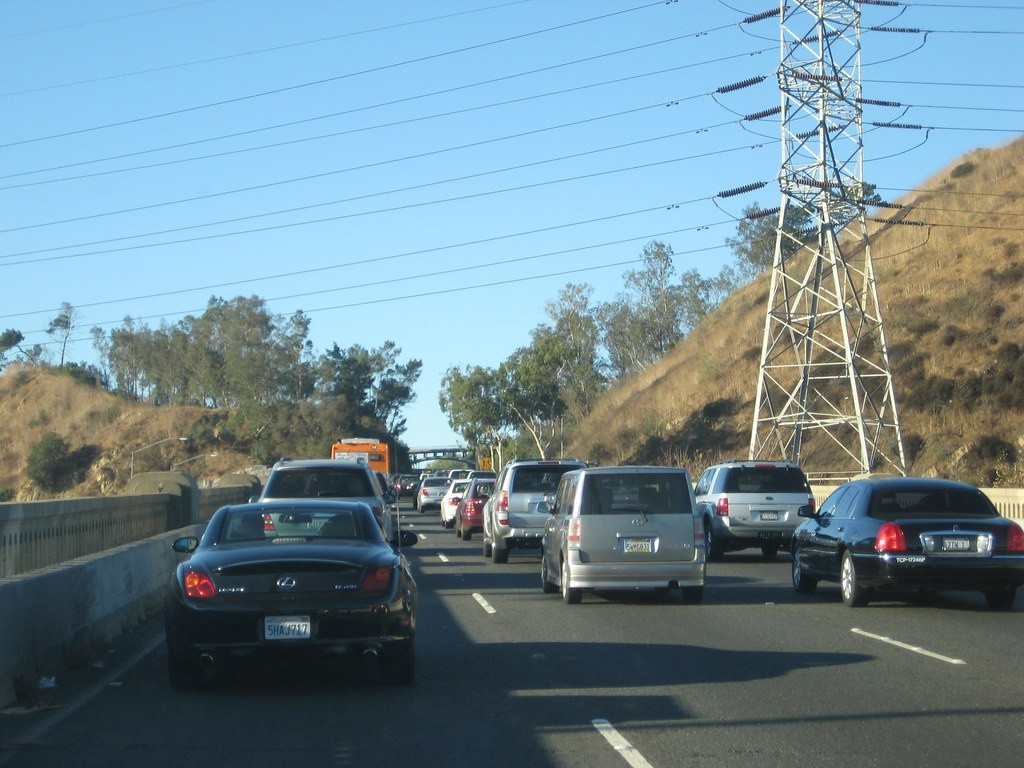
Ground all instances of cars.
[397,474,421,498]
[455,477,504,542]
[416,476,456,515]
[439,477,474,528]
[169,500,418,684]
[789,476,1024,606]
[536,466,708,607]
[374,467,497,502]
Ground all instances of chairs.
[228,523,264,541]
[319,520,354,539]
[595,487,615,512]
[638,486,665,503]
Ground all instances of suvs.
[692,457,818,560]
[248,457,397,547]
[481,458,591,567]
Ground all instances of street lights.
[131,437,188,482]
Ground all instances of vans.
[334,436,390,483]
